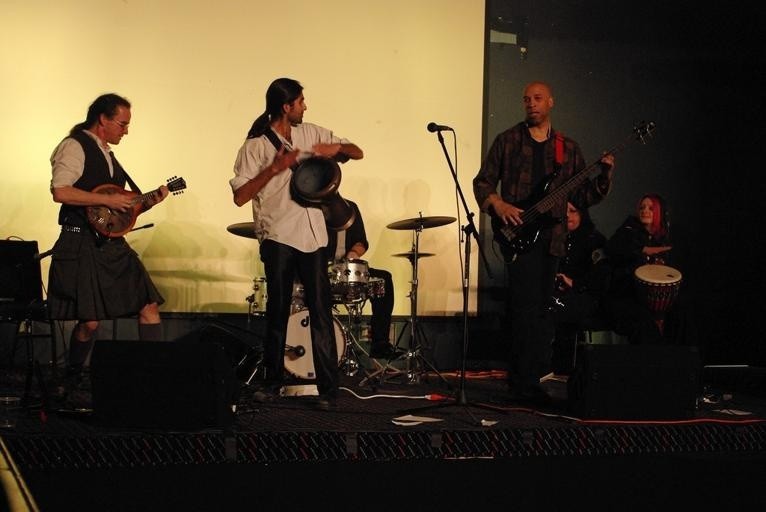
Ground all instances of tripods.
[346,235,510,426]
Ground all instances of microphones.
[427,122,453,133]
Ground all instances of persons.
[542,196,614,390]
[41,92,174,405]
[603,193,678,344]
[312,194,419,363]
[231,76,364,412]
[472,81,618,406]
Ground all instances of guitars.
[492,121,657,255]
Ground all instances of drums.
[326,259,368,294]
[282,310,347,379]
[636,265,682,335]
[289,155,357,231]
[253,276,303,313]
[357,276,386,298]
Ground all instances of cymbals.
[386,217,454,230]
[228,222,259,239]
[392,253,432,260]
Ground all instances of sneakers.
[252,385,281,403]
[314,393,337,410]
